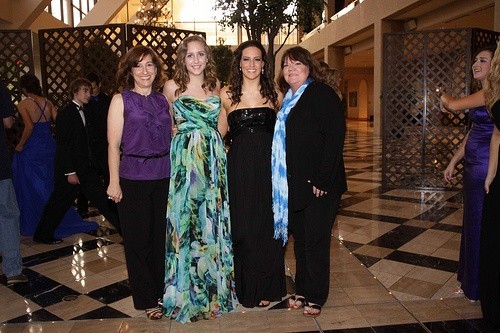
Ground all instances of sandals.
[304,303,321,317]
[257,300,270,307]
[287,294,307,310]
[146,308,162,320]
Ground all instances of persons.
[315,61,347,118]
[12,74,60,238]
[278,46,347,317]
[172,40,285,308]
[74,72,113,219]
[439,46,497,303]
[31,78,121,244]
[0,81,29,283]
[162,37,235,322]
[106,46,175,320]
[481,41,500,333]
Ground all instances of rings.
[108,196,112,199]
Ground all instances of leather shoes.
[33,234,63,244]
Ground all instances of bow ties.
[74,102,85,111]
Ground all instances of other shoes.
[7,274,28,283]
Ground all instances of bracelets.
[18,143,25,147]
